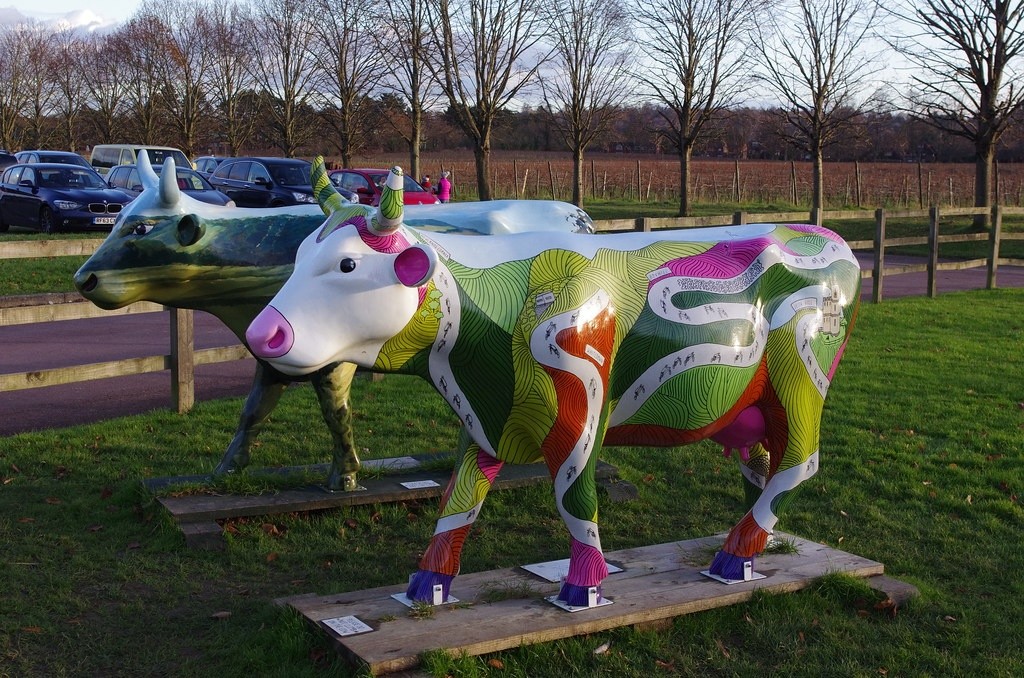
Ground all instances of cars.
[0,149,19,178]
[191,157,226,183]
[12,151,102,177]
[208,156,360,209]
[100,164,237,213]
[328,168,441,208]
[0,162,141,236]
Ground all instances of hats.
[422,175,430,182]
[441,171,450,178]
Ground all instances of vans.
[89,144,196,181]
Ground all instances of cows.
[71,148,862,608]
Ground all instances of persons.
[437,171,451,203]
[421,175,432,191]
[379,176,387,188]
